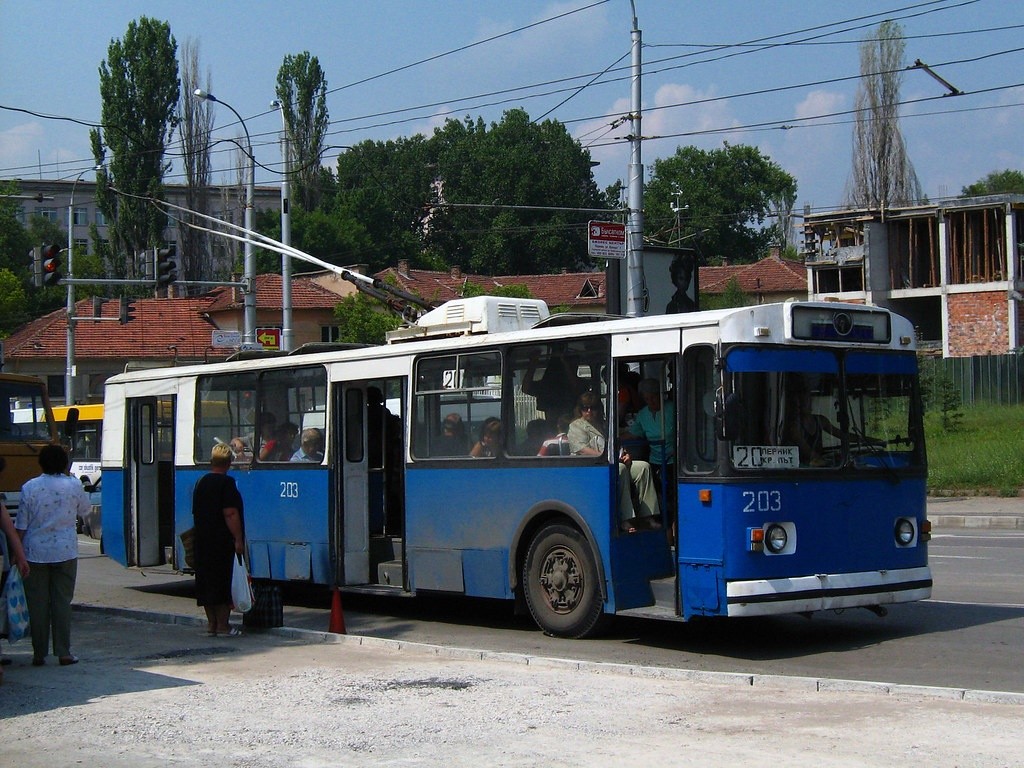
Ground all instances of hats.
[212,444,232,459]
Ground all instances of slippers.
[206,631,217,636]
[217,629,245,638]
[33,659,46,666]
[59,656,79,666]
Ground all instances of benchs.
[547,433,660,497]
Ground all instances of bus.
[10,400,230,535]
[0,373,82,575]
[101,296,933,643]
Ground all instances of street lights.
[193,89,258,347]
[268,100,293,350]
[65,164,107,402]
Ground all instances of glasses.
[580,404,598,412]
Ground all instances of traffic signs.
[254,327,281,349]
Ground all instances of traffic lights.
[40,244,61,288]
[153,246,177,291]
[28,247,43,287]
[94,295,109,324]
[119,294,136,326]
[139,250,155,289]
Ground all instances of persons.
[230,412,324,464]
[193,443,245,637]
[0,458,30,576]
[15,445,91,665]
[666,255,695,315]
[344,350,885,530]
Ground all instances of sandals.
[643,518,661,528]
[619,521,637,534]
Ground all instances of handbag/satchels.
[0,565,30,644]
[180,525,195,569]
[231,552,255,613]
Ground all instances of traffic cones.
[327,587,348,635]
